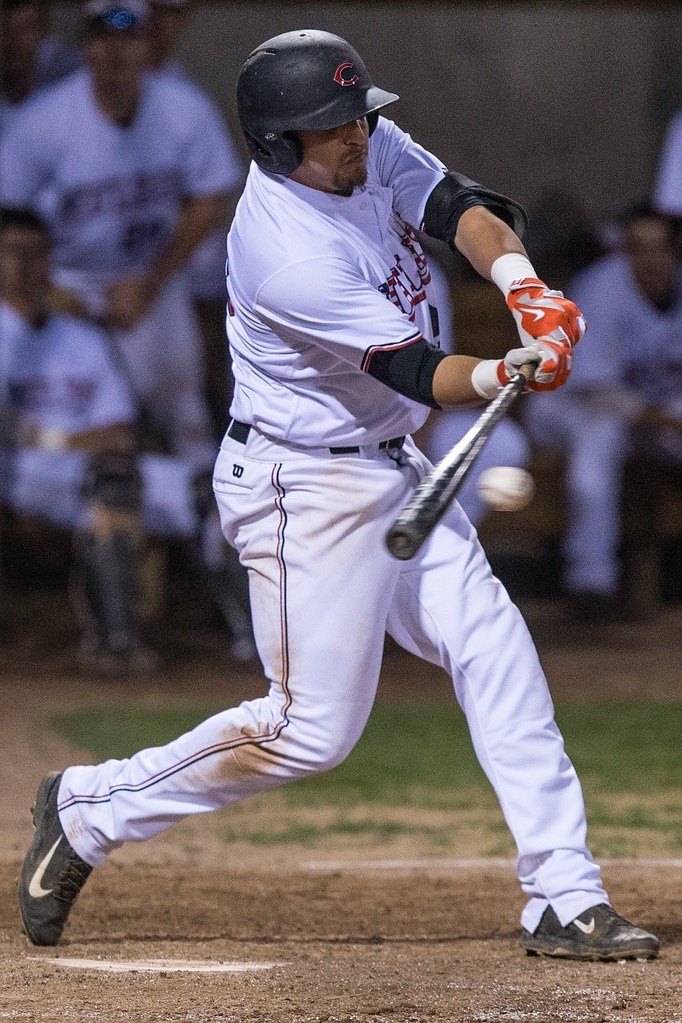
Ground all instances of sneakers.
[520,904,660,963]
[14,771,93,946]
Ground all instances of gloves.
[470,336,572,399]
[491,253,588,348]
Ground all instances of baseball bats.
[383,355,540,562]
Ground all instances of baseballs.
[477,466,536,513]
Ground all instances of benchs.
[417,286,682,622]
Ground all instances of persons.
[12,25,662,963]
[0,1,534,680]
[521,110,682,621]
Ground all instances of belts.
[229,419,410,454]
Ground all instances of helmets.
[236,28,400,175]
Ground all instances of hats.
[80,1,154,32]
